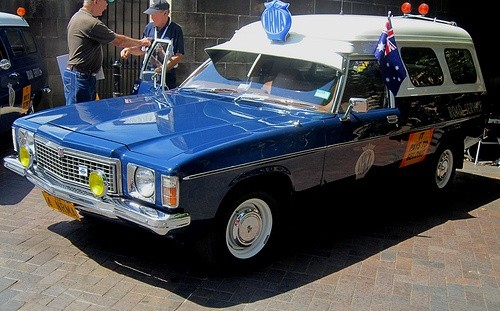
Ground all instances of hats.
[143,0,169,15]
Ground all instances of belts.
[68,65,97,76]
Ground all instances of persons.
[120,0,184,91]
[63,0,152,105]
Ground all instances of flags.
[373,15,407,96]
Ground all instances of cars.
[3,0,490,273]
